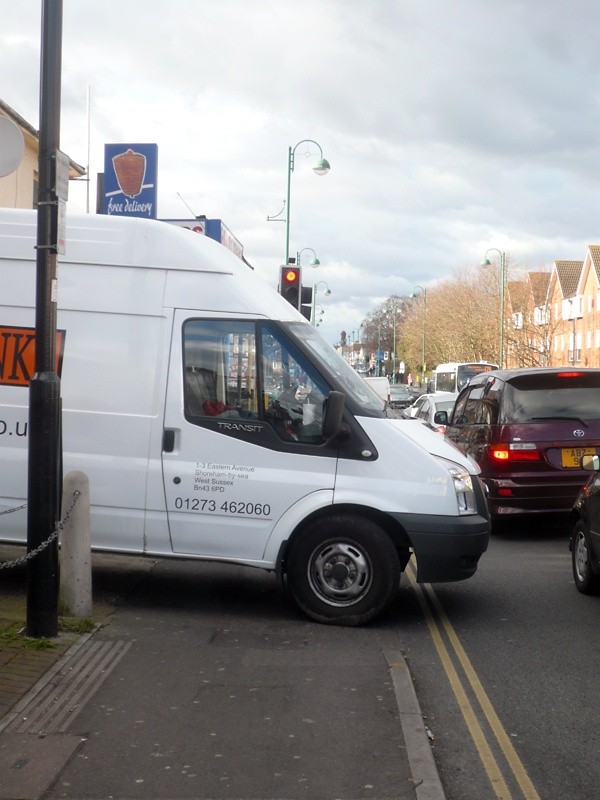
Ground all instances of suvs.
[434,368,600,534]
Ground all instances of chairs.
[185,369,218,414]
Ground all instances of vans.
[0,206,491,626]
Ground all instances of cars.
[570,454,600,596]
[355,376,458,429]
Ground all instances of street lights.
[360,319,367,372]
[481,248,506,372]
[296,247,320,268]
[312,281,331,326]
[408,286,426,384]
[369,315,379,376]
[383,306,397,383]
[285,140,330,388]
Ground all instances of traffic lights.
[279,265,312,322]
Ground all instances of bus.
[431,360,499,395]
